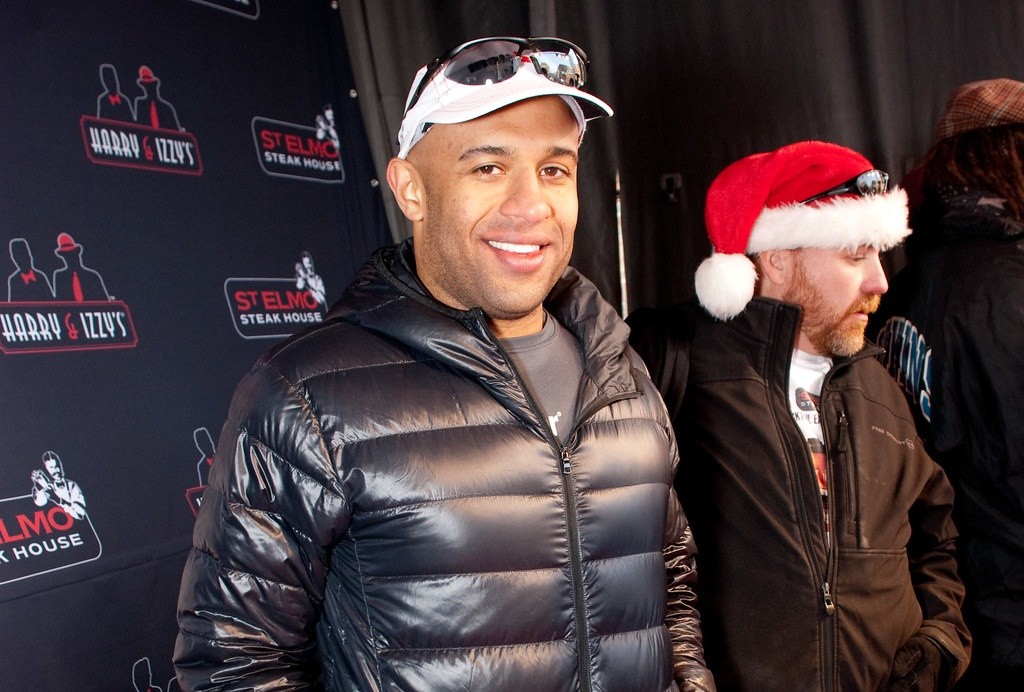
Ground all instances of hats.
[396,37,614,161]
[694,140,911,322]
[933,78,1024,135]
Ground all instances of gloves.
[885,636,955,692]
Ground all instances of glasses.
[395,38,587,147]
[793,169,890,207]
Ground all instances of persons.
[173,35,713,692]
[620,141,972,692]
[879,76,1024,692]
